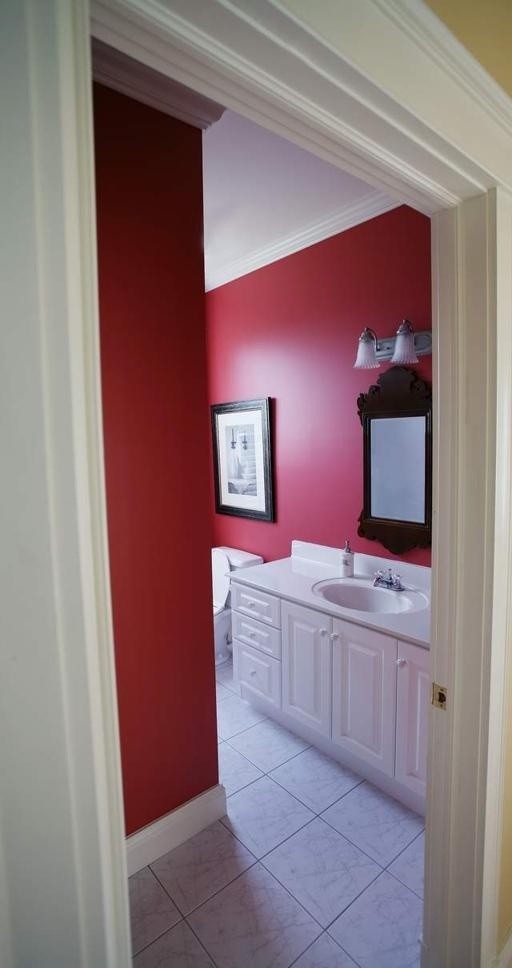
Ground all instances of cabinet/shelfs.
[231,579,429,819]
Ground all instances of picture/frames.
[210,397,276,522]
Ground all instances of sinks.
[312,577,430,614]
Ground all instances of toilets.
[212,546,263,666]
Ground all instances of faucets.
[374,576,393,589]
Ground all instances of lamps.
[353,319,432,369]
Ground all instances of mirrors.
[357,365,432,555]
